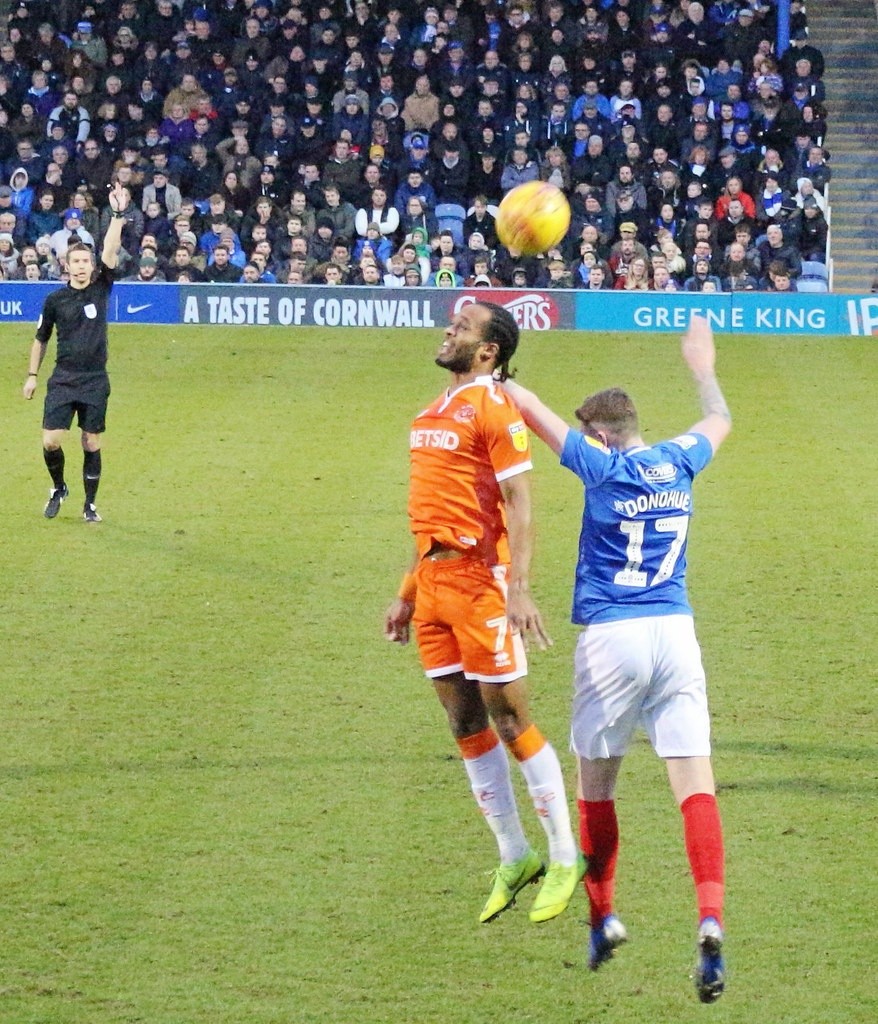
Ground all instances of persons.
[492,312,734,1007]
[22,180,126,524]
[384,300,587,923]
[0,0,829,294]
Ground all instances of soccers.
[494,181,571,258]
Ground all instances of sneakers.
[694,916,725,1003]
[530,851,587,923]
[44,482,69,518]
[589,915,625,971]
[83,502,102,523]
[479,848,546,924]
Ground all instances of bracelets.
[397,572,418,602]
[29,373,37,376]
